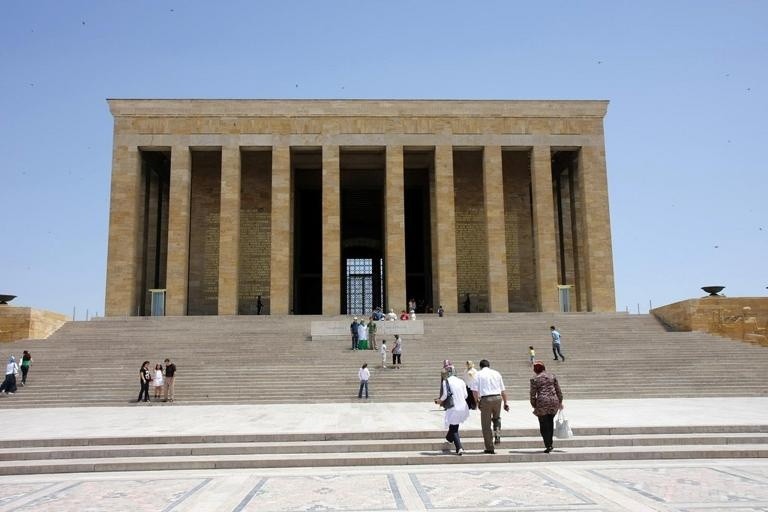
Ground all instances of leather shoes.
[495,439,500,444]
[483,449,495,453]
[544,446,553,453]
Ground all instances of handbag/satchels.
[463,387,477,410]
[439,394,454,410]
[554,418,573,439]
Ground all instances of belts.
[481,393,500,398]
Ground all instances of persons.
[439,359,457,410]
[0,356,18,395]
[357,319,368,350]
[381,339,389,369]
[350,317,358,350]
[463,293,471,312]
[367,315,378,350]
[528,345,535,364]
[463,359,479,409]
[372,297,417,321]
[153,363,165,400]
[162,358,177,402]
[256,296,265,315]
[550,325,565,362]
[438,305,444,317]
[357,362,370,400]
[136,362,150,403]
[529,360,563,452]
[392,335,401,370]
[144,361,152,401]
[472,360,510,454]
[19,350,34,385]
[435,367,469,455]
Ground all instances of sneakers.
[457,449,463,455]
[447,439,452,444]
